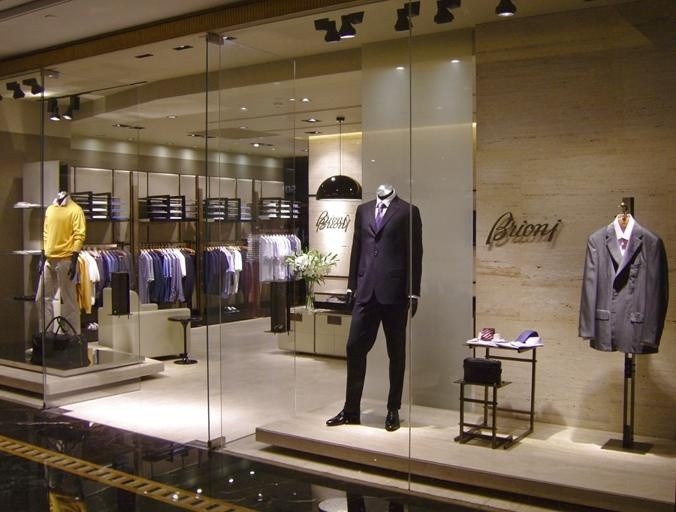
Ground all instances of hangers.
[258,230,300,246]
[613,202,639,232]
[78,240,245,258]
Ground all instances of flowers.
[284,247,340,303]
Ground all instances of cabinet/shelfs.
[204,196,251,220]
[69,188,125,220]
[10,202,47,301]
[260,196,301,220]
[147,194,197,222]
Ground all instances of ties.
[481,328,495,340]
[515,329,539,342]
[375,202,385,233]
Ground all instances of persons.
[327,184,423,431]
[25,191,86,355]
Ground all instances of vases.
[302,274,315,311]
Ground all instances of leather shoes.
[386,409,399,431]
[326,410,360,426]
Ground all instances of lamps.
[315,122,362,201]
[314,0,517,46]
[7,79,81,123]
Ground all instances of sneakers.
[223,306,240,314]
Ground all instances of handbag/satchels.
[31,331,91,369]
[463,357,502,385]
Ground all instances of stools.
[169,315,202,366]
[447,378,511,449]
